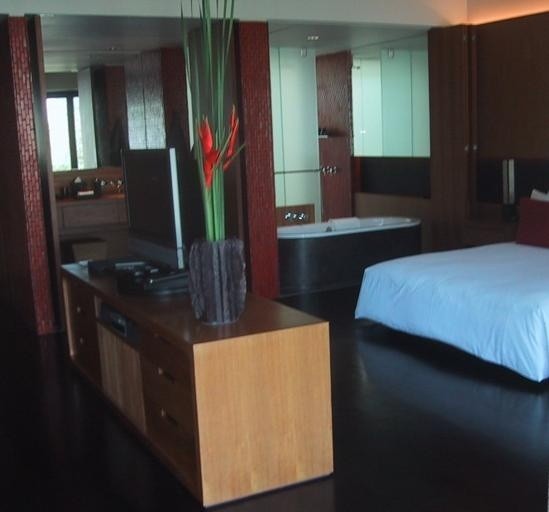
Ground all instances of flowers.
[179,1,246,243]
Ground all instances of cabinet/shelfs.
[54,194,133,235]
[60,259,333,509]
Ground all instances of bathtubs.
[276,215,423,298]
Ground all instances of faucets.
[298,213,306,223]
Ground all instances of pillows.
[516,197,549,248]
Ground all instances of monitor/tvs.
[120,147,185,283]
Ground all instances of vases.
[189,241,249,326]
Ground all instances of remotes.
[113,261,144,267]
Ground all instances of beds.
[354,157,548,389]
